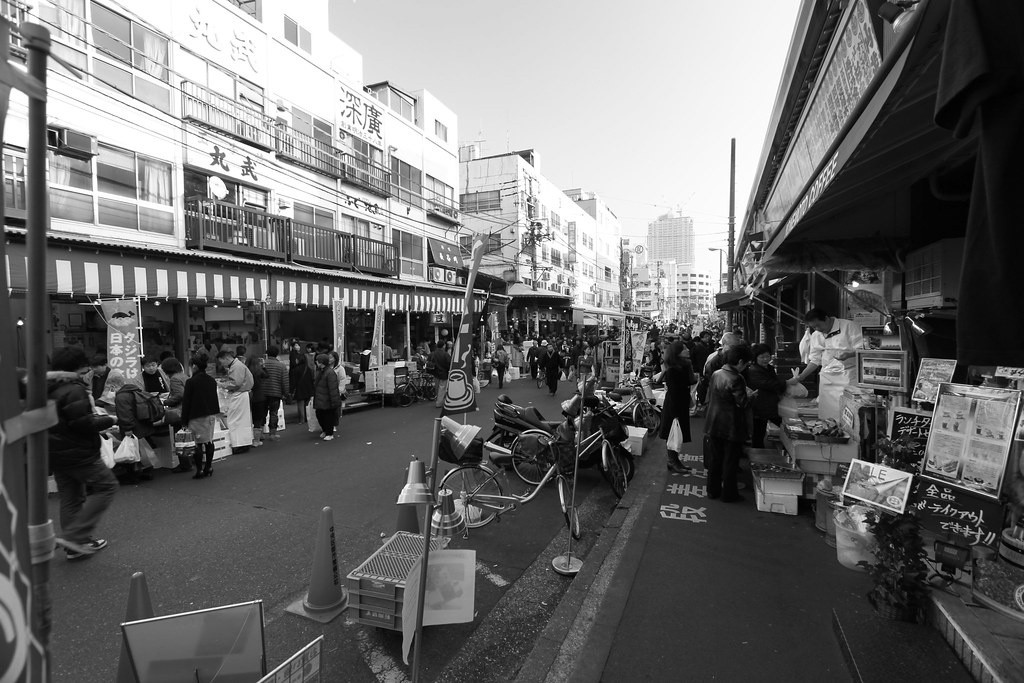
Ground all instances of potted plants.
[854,513,924,622]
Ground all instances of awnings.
[716,257,902,323]
[3,225,489,315]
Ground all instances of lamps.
[431,488,465,535]
[883,321,894,337]
[760,220,781,233]
[741,262,760,267]
[745,250,766,259]
[750,240,766,249]
[396,461,432,506]
[933,538,972,576]
[441,416,482,462]
[904,316,934,339]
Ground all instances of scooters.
[561,364,662,431]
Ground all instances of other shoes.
[721,493,744,501]
[709,491,720,499]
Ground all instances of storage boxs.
[780,422,862,463]
[347,530,451,635]
[364,361,417,395]
[509,366,520,381]
[754,484,798,515]
[752,463,802,496]
[200,430,233,463]
[625,425,649,456]
[263,399,285,433]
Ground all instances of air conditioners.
[332,128,349,151]
[287,236,304,255]
[445,269,457,286]
[569,276,576,285]
[248,226,277,251]
[458,276,467,286]
[61,127,99,159]
[557,273,565,283]
[452,209,461,222]
[428,266,445,281]
[345,249,366,267]
[541,271,551,281]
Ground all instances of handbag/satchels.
[503,371,512,383]
[560,371,566,381]
[491,368,498,379]
[306,401,322,433]
[341,390,349,400]
[666,418,684,453]
[113,434,140,463]
[790,366,809,399]
[426,352,436,373]
[99,432,116,468]
[473,378,480,394]
[175,426,196,457]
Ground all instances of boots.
[666,450,692,470]
[667,450,689,474]
[251,428,263,447]
[269,428,280,441]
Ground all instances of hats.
[52,345,89,371]
[541,340,548,346]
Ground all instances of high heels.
[204,467,213,477]
[193,470,204,479]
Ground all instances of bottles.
[942,411,951,429]
[953,411,965,433]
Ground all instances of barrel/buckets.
[997,527,1024,576]
[825,495,850,549]
[814,489,836,533]
[832,511,880,573]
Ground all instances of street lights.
[708,247,723,293]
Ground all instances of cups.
[977,428,981,434]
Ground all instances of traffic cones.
[382,502,422,547]
[112,572,155,683]
[284,506,349,623]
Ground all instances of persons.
[214,351,253,454]
[747,343,797,443]
[244,355,269,448]
[796,308,874,461]
[360,343,371,383]
[659,340,698,475]
[180,354,220,479]
[576,344,593,394]
[540,343,564,395]
[83,355,125,446]
[161,357,193,472]
[260,346,291,439]
[195,335,219,362]
[106,368,149,483]
[492,344,509,388]
[380,324,760,503]
[234,346,246,365]
[22,347,120,559]
[431,341,451,408]
[132,355,180,476]
[158,351,184,375]
[289,342,346,440]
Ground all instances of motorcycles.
[478,365,634,484]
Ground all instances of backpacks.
[130,390,165,424]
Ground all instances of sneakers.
[320,432,326,439]
[55,535,95,554]
[324,435,334,441]
[67,539,107,559]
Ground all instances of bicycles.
[438,421,581,541]
[608,385,658,438]
[510,403,629,500]
[535,366,547,389]
[394,372,437,407]
